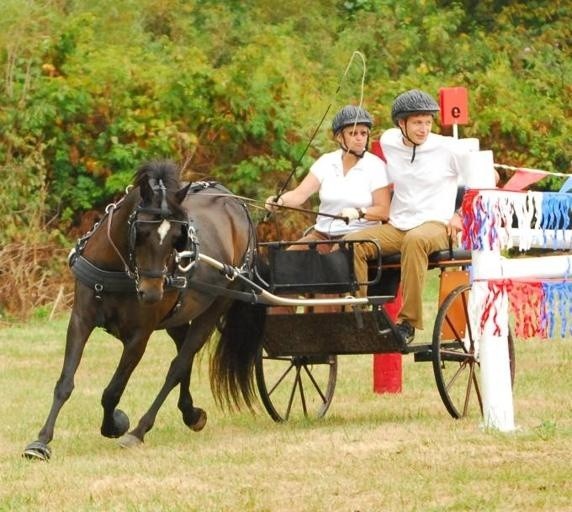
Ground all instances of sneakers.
[395,321,415,344]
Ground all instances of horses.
[19,158,269,463]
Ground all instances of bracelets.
[455,210,465,219]
[279,197,284,210]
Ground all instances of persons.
[264,105,392,314]
[330,89,483,343]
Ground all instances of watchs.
[357,206,367,219]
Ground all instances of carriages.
[17,159,515,462]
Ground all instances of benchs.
[368,249,471,342]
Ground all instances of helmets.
[332,106,372,135]
[391,89,440,125]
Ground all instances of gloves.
[337,208,359,220]
